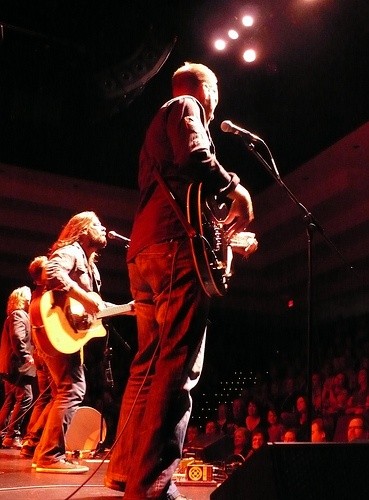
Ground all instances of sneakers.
[32,458,89,473]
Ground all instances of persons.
[0,257,50,456]
[96,354,369,472]
[103,63,258,500]
[32,211,136,474]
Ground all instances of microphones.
[220,119,259,141]
[108,230,131,243]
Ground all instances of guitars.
[186,181,234,298]
[29,289,135,358]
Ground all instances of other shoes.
[2,442,21,450]
[175,494,192,500]
[103,475,127,492]
[20,440,34,457]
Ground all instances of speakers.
[196,432,235,462]
[210,441,369,500]
